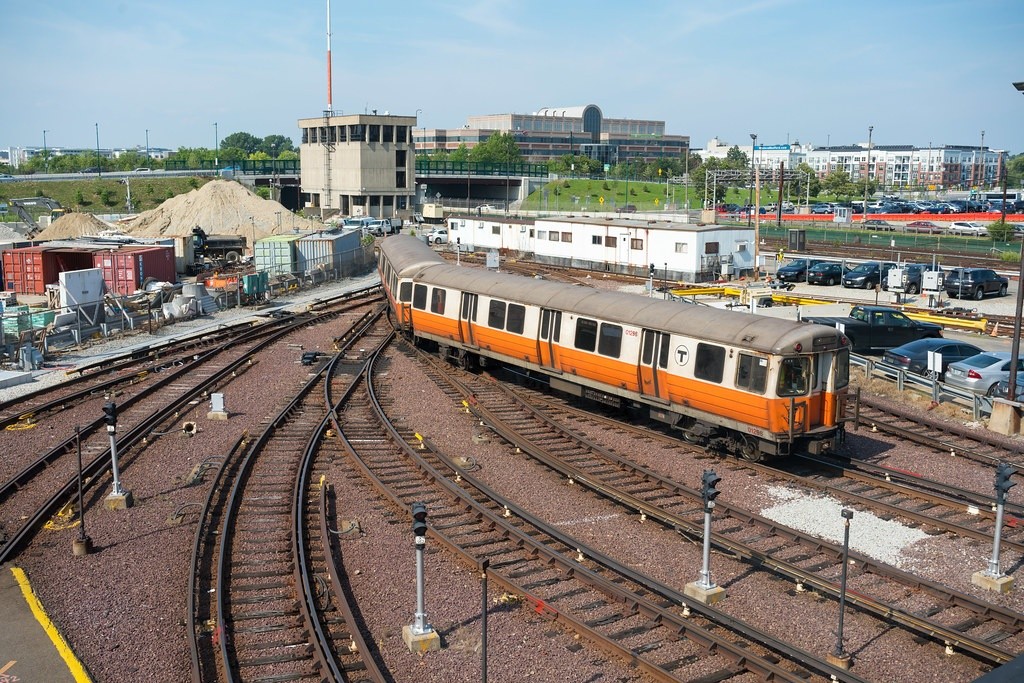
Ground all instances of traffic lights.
[413,506,427,549]
[998,464,1019,500]
[101,403,116,434]
[700,471,721,511]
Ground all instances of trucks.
[342,217,403,236]
[191,233,245,261]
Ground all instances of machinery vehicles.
[8,197,72,240]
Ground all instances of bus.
[971,193,1021,203]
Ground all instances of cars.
[476,203,495,210]
[426,230,448,244]
[0,175,20,183]
[777,258,1009,300]
[880,338,1024,406]
[716,198,1024,235]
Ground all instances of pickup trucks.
[800,306,944,355]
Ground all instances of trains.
[377,236,862,462]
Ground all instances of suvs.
[616,205,637,213]
[79,166,102,173]
[220,166,240,170]
[131,168,151,173]
